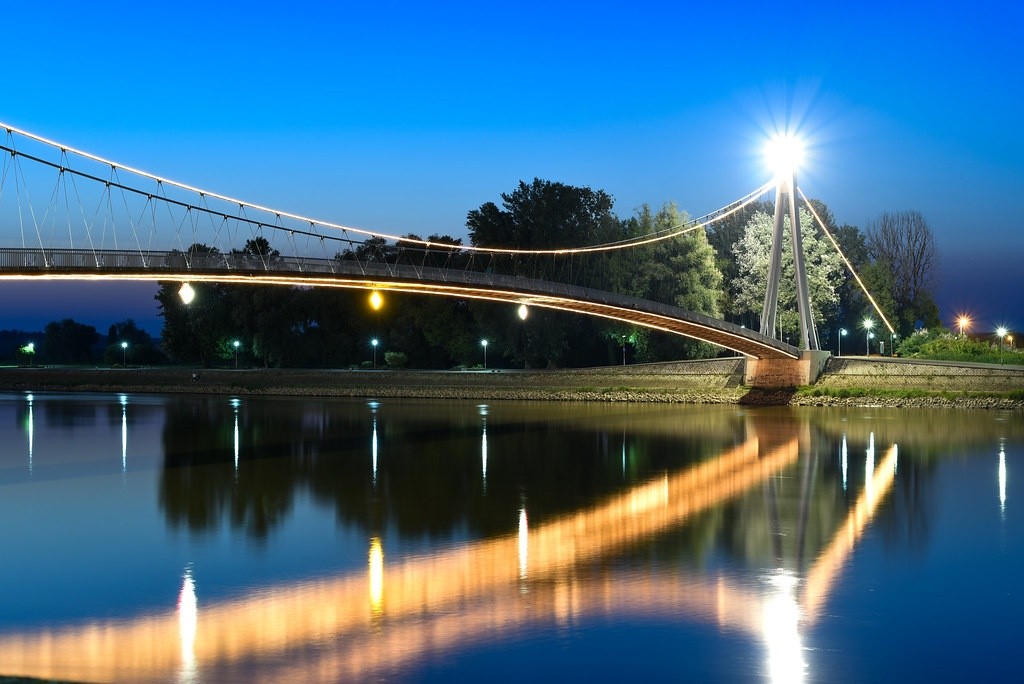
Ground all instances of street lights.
[482,339,487,371]
[839,328,847,356]
[997,327,1006,366]
[234,341,239,370]
[864,320,874,357]
[372,340,377,368]
[122,342,127,367]
